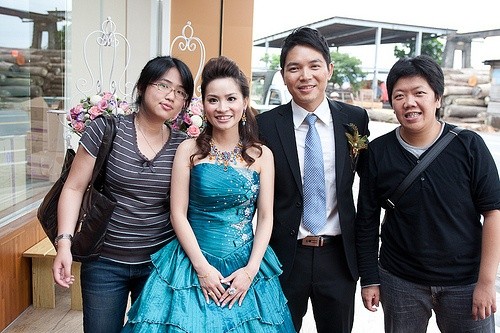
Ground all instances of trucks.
[248,84,292,116]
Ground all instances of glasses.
[143,82,189,100]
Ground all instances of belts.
[296,236,344,249]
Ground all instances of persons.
[354,56,500,333]
[52,56,194,333]
[121,57,297,333]
[253,27,370,333]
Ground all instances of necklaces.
[137,120,164,155]
[207,139,247,166]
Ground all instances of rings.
[227,288,236,296]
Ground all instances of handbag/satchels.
[37,114,117,264]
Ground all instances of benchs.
[20,237,82,312]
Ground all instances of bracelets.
[242,266,252,284]
[197,265,214,279]
[55,233,74,251]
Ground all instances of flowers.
[170,96,205,139]
[344,123,368,170]
[66,93,134,133]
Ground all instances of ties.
[301,113,327,235]
